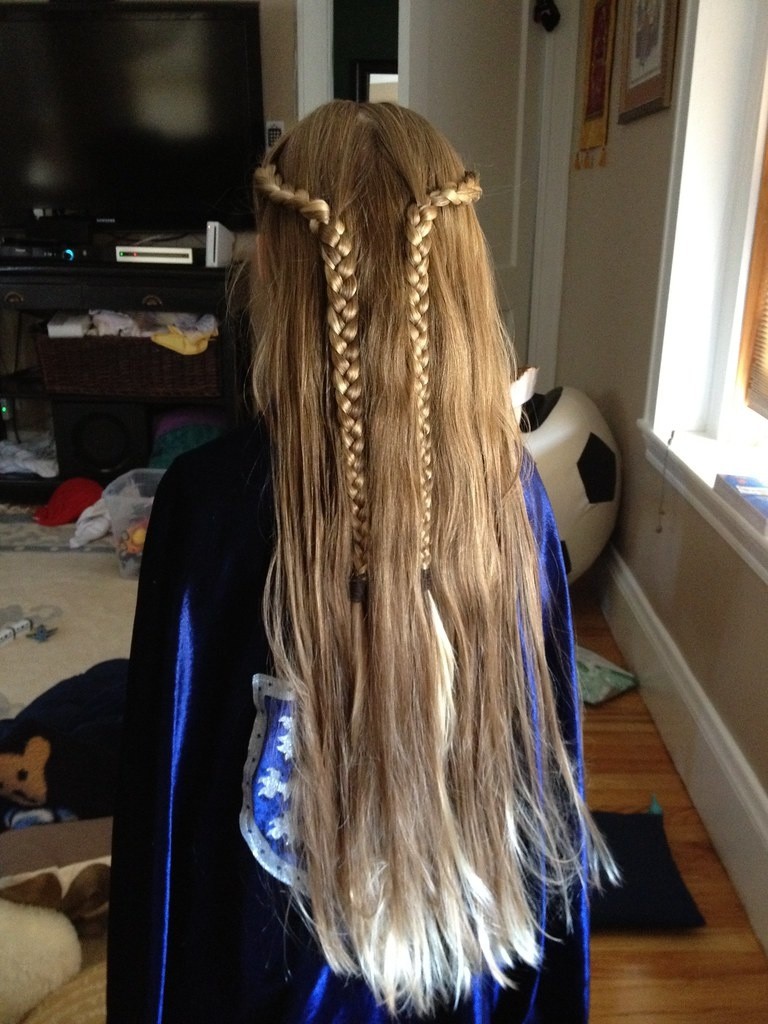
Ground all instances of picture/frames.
[618,0,678,123]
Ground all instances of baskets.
[31,317,222,397]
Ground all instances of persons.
[105,101,620,1024]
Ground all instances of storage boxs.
[103,468,166,577]
[29,319,220,398]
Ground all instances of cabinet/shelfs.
[0,275,251,503]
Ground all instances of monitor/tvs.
[0,0,267,231]
